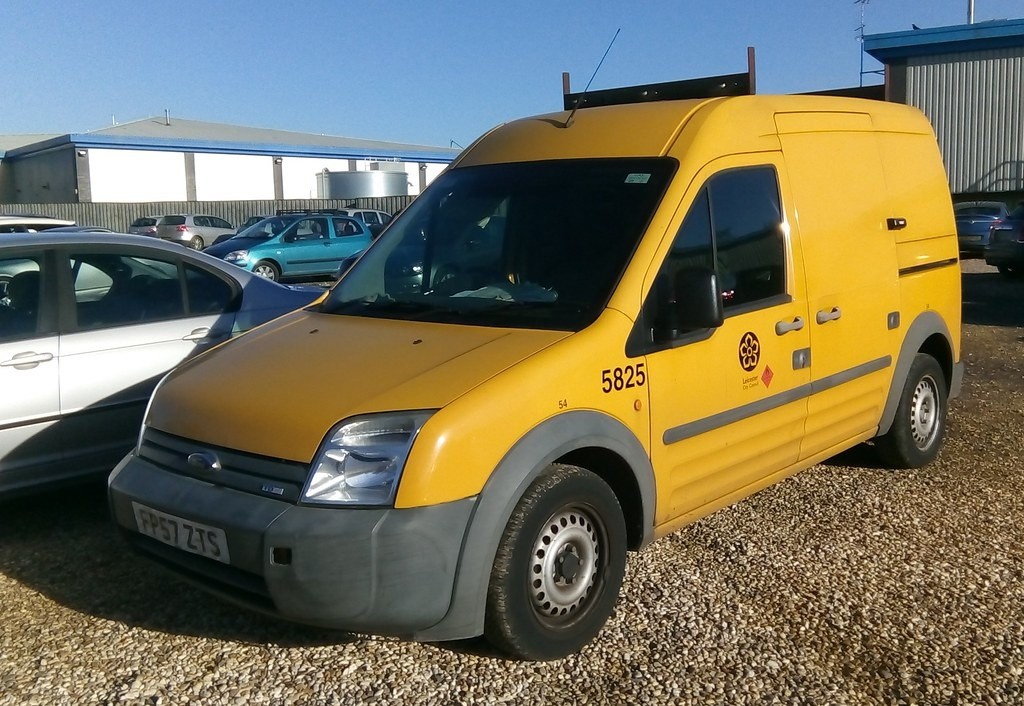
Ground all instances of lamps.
[78,151,86,157]
[275,158,283,165]
[420,165,427,171]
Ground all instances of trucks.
[107,95,965,662]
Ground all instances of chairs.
[0,269,40,336]
[344,225,353,235]
[307,223,326,239]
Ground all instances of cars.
[202,211,373,286]
[952,199,1023,279]
[335,208,405,242]
[0,230,334,520]
[130,214,237,251]
